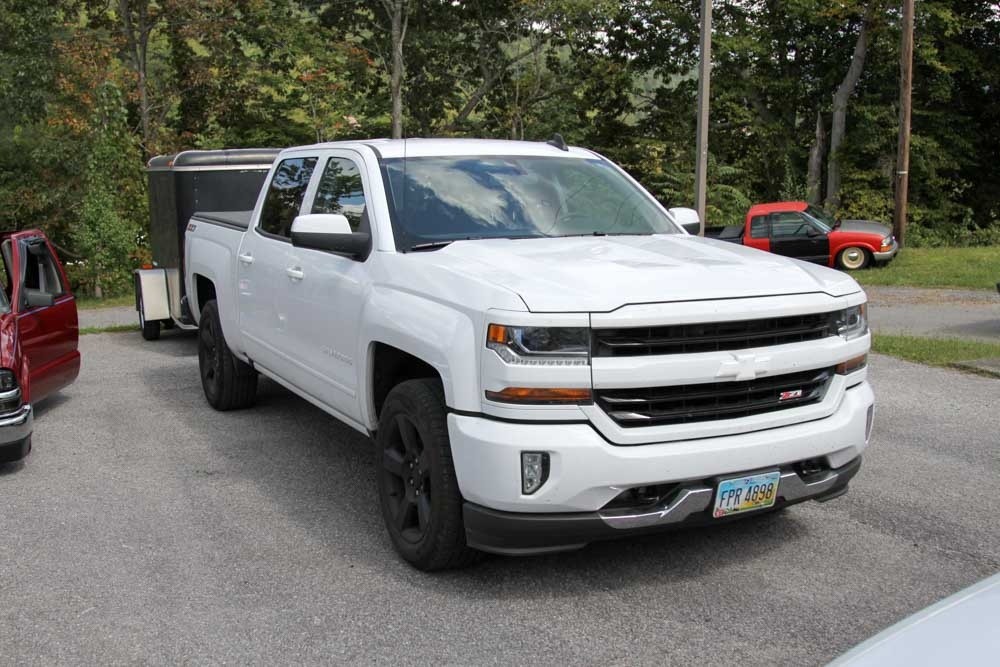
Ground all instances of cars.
[0,229,81,461]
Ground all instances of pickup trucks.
[179,131,877,571]
[704,200,899,271]
